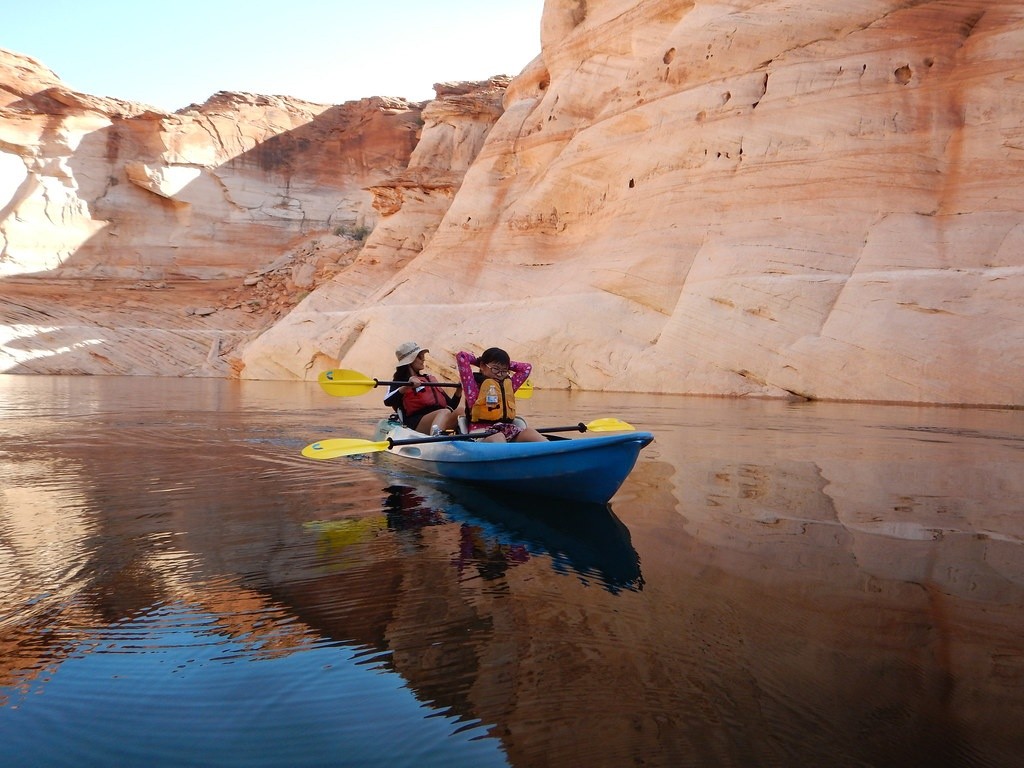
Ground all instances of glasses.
[484,363,510,377]
[418,354,424,359]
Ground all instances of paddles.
[301,417,636,460]
[318,369,533,399]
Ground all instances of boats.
[385,465,646,596]
[374,412,654,507]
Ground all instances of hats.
[395,342,429,367]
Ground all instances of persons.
[456,348,550,442]
[384,341,466,435]
